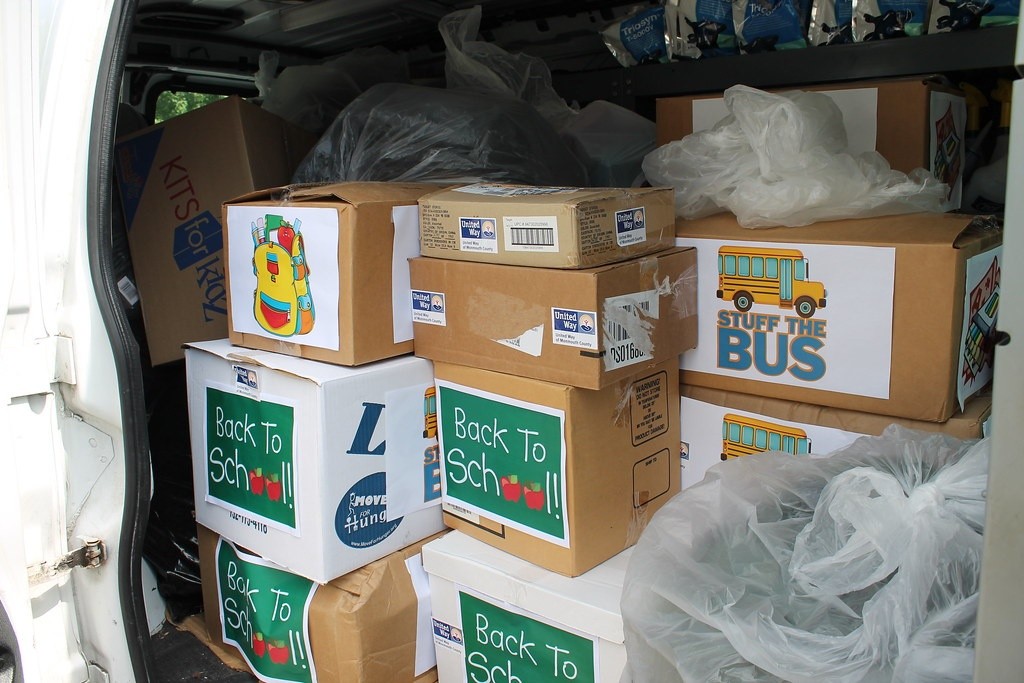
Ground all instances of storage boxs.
[130,77,1004,683]
